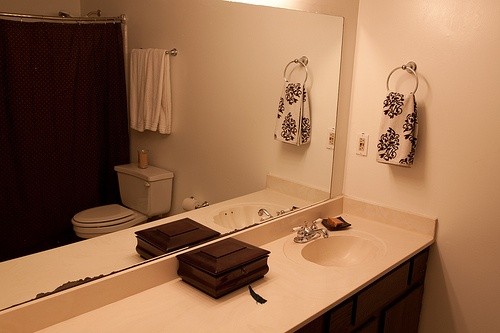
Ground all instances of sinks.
[212,204,276,230]
[300,234,388,269]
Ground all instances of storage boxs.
[134,217,221,259]
[176,237,271,299]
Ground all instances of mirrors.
[0,0,358,333]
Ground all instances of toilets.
[71,161,175,239]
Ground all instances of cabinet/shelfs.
[291,247,430,333]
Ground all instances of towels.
[128,47,172,135]
[273,82,312,146]
[375,91,419,169]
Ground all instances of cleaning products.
[138,149,149,169]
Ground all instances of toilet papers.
[181,197,198,210]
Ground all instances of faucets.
[303,224,329,243]
[257,208,272,221]
